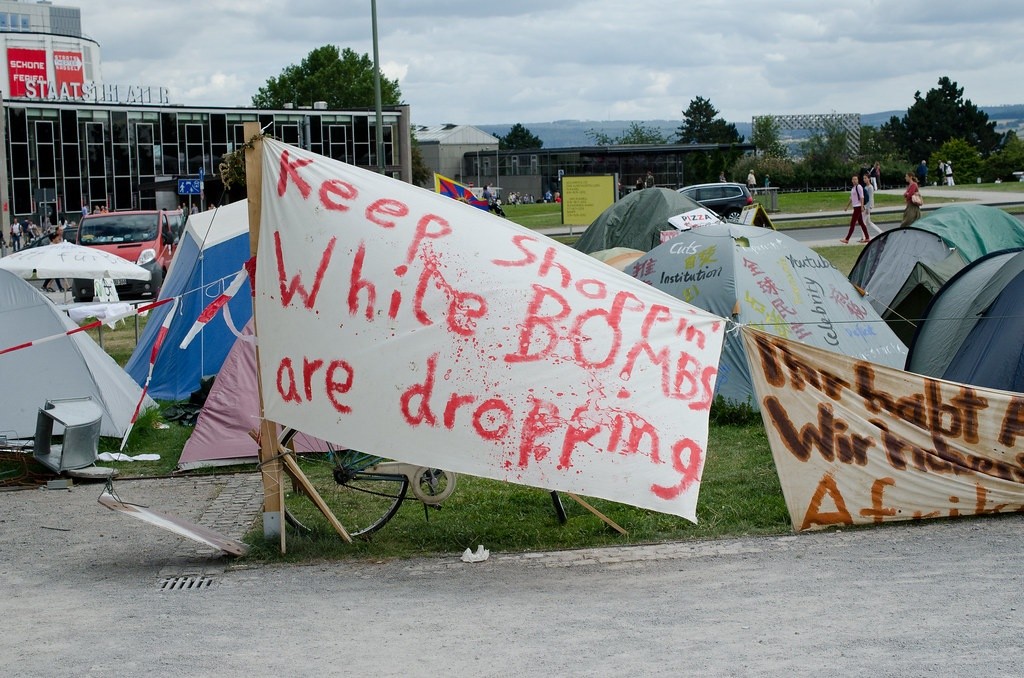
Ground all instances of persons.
[507,190,560,205]
[483,182,494,206]
[840,174,883,243]
[747,170,756,188]
[765,174,770,188]
[917,160,927,187]
[636,172,654,190]
[900,172,922,227]
[858,163,882,190]
[935,160,955,186]
[719,172,726,182]
[40,227,72,291]
[176,203,216,218]
[0,204,108,257]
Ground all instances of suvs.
[674,182,755,223]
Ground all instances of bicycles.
[277,424,568,541]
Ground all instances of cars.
[11,208,186,304]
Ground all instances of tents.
[908,248,1024,391]
[123,198,252,402]
[623,223,908,409]
[571,188,727,254]
[0,269,156,441]
[179,314,350,464]
[849,204,1024,349]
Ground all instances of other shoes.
[839,238,849,244]
[67,287,73,291]
[857,239,864,242]
[41,286,48,292]
[861,240,870,243]
[47,287,55,292]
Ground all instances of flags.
[435,173,487,209]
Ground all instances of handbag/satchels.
[911,192,923,205]
[856,184,869,205]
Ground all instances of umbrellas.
[0,239,152,305]
